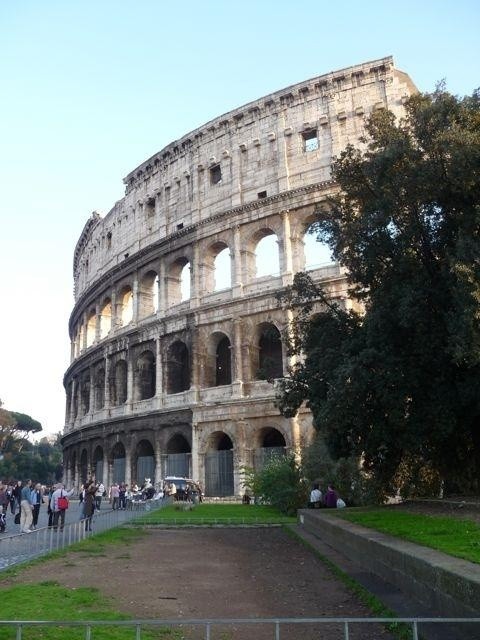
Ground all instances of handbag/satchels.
[58,498,68,509]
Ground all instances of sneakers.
[23,529,33,534]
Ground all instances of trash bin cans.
[189,489,199,505]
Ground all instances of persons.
[0,478,176,537]
[242,489,252,505]
[307,482,323,509]
[322,484,340,509]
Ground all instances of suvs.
[125,476,205,511]
